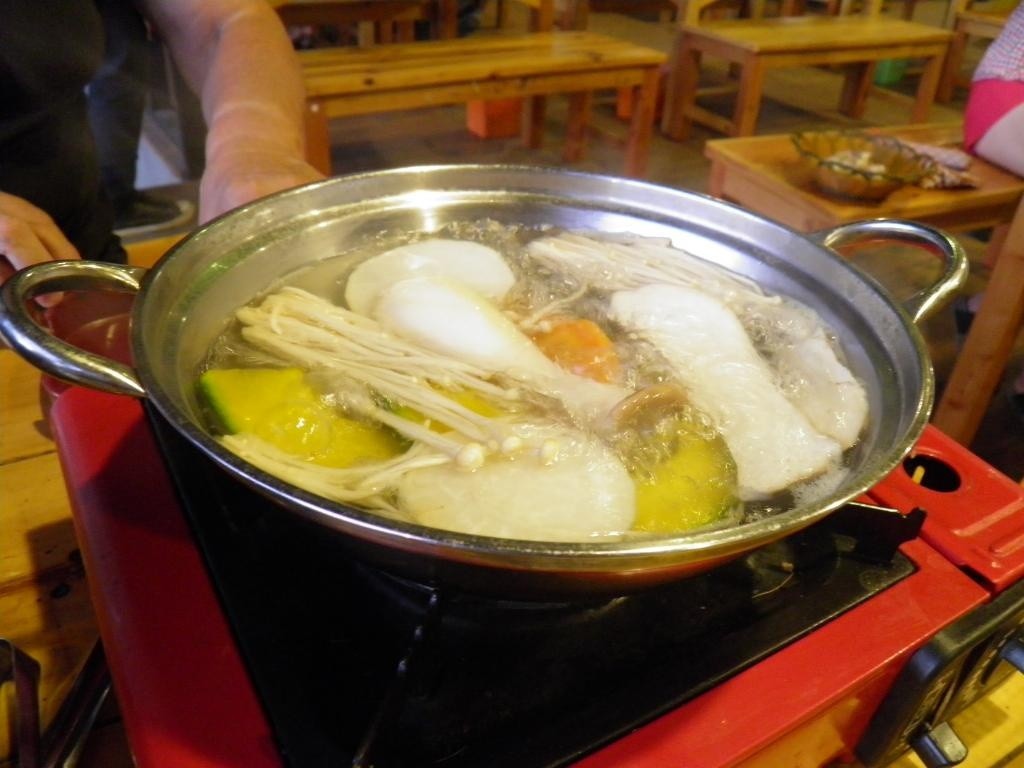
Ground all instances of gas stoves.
[49,375,1023,768]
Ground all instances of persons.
[0,0,327,308]
[954,0,1024,342]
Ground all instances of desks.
[698,119,1024,262]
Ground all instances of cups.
[45,287,134,398]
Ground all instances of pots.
[0,162,970,603]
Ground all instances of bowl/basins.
[788,125,939,205]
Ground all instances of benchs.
[290,26,673,183]
[657,13,965,145]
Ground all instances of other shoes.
[1005,383,1024,431]
[954,293,977,345]
